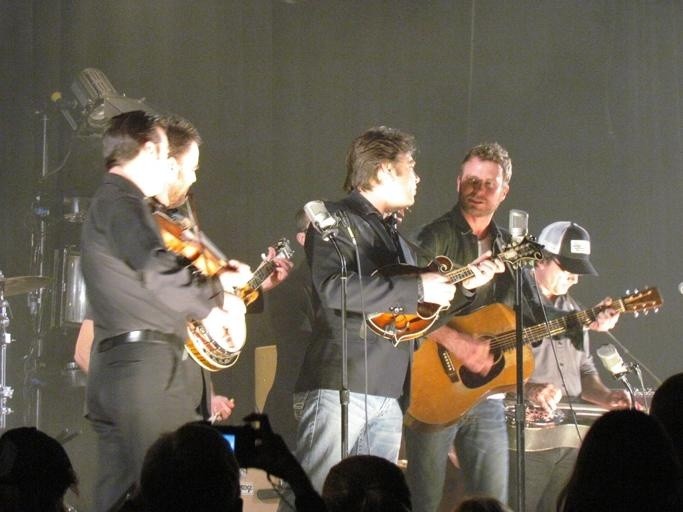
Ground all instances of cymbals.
[1,276,48,296]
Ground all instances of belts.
[99,331,179,353]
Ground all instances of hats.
[538,220,597,275]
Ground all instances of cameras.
[212,425,254,466]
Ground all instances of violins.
[154,210,260,303]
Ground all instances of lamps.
[64,67,126,132]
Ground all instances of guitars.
[184,238,294,371]
[404,285,661,431]
[364,235,545,346]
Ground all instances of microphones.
[303,197,339,240]
[506,208,530,243]
[594,340,628,378]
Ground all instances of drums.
[47,249,88,328]
